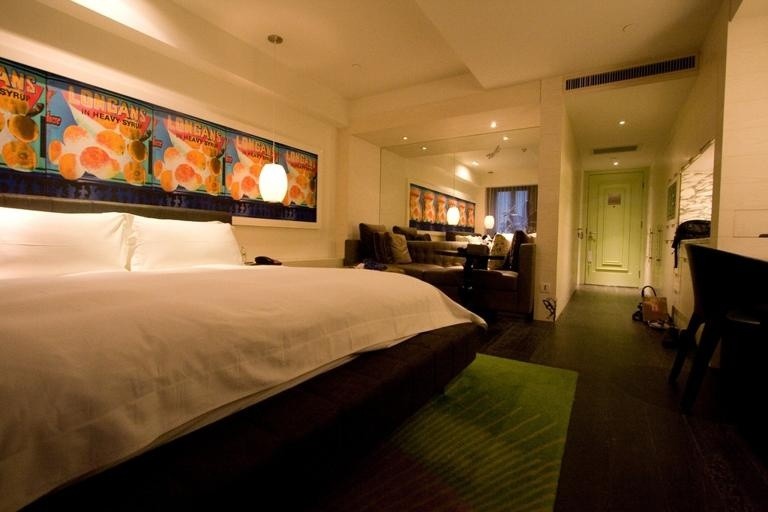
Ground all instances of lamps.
[259,34,288,203]
[447,153,460,230]
[484,171,495,229]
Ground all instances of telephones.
[255,256,282,265]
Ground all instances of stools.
[344,239,535,316]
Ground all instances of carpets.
[326,352,579,512]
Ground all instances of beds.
[0,194,489,512]
[447,231,483,242]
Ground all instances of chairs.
[670,243,767,409]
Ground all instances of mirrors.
[379,126,537,241]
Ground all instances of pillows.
[126,214,245,271]
[487,234,512,270]
[509,230,535,269]
[360,222,412,265]
[0,206,131,277]
[393,225,431,240]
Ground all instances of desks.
[435,249,505,307]
